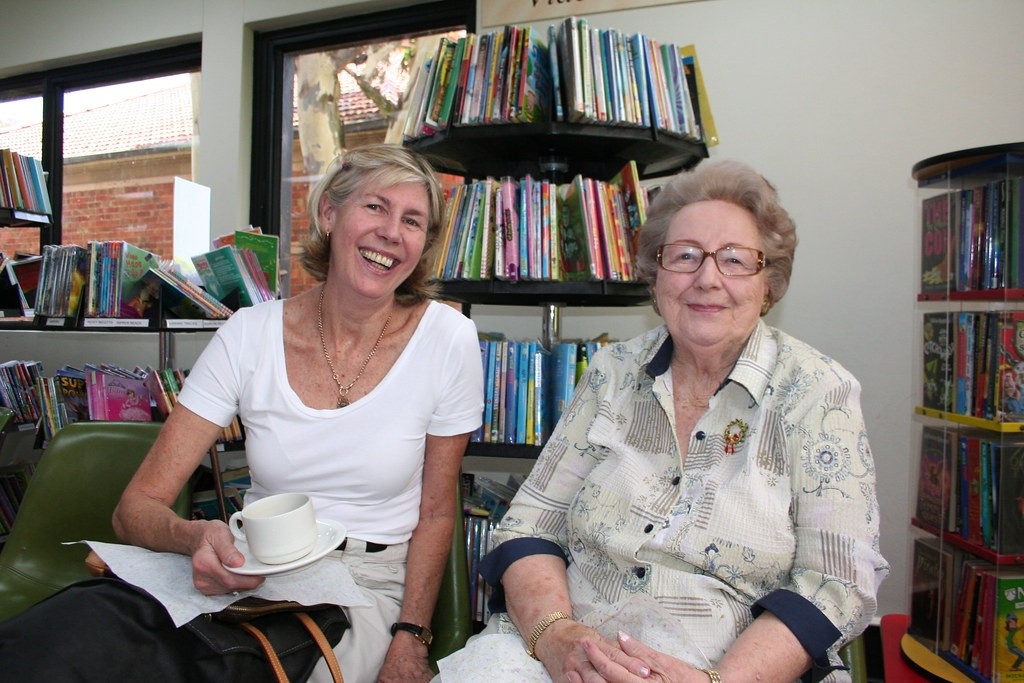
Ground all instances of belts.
[337,539,387,554]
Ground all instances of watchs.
[391,623,433,644]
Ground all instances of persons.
[0,143,484,683]
[431,161,889,683]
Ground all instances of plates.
[222,518,346,575]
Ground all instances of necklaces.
[319,281,392,407]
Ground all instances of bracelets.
[701,668,721,683]
[526,611,569,662]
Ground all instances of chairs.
[425,453,476,670]
[0,400,199,620]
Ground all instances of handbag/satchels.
[86,551,352,683]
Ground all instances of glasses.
[653,244,776,277]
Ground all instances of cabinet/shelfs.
[407,21,735,503]
[888,138,1024,679]
[0,304,250,527]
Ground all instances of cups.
[229,493,316,565]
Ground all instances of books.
[194,465,250,524]
[909,177,1024,683]
[405,14,719,624]
[0,149,280,445]
